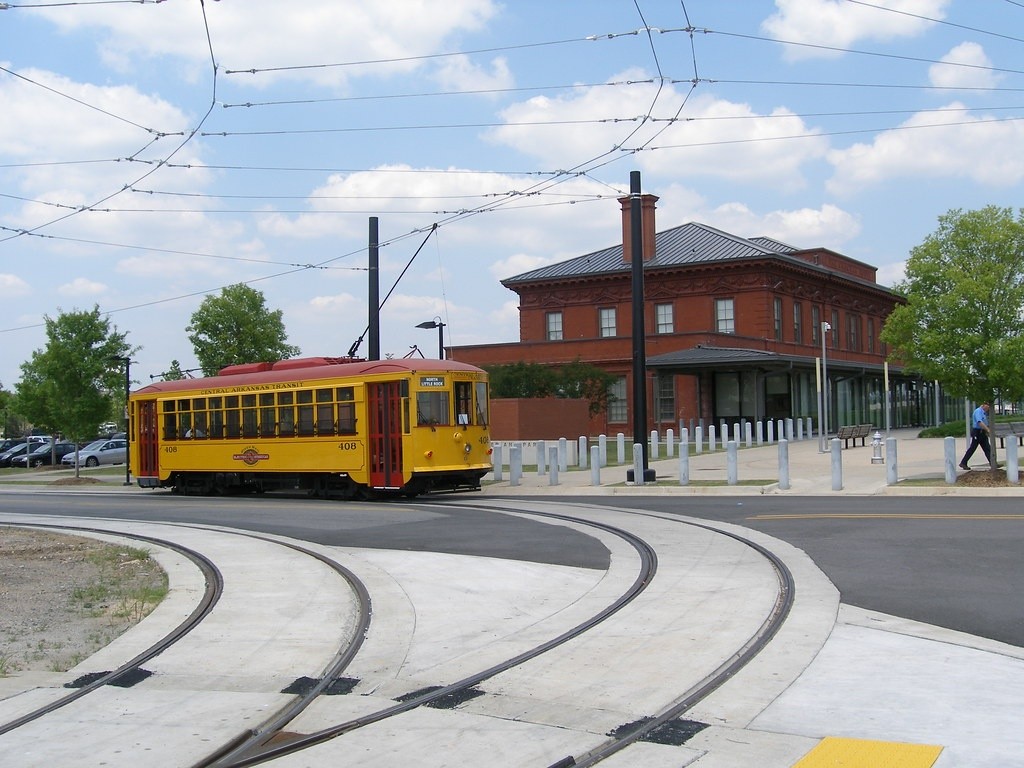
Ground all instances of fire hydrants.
[870,430,885,464]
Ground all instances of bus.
[128,354,493,502]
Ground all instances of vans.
[0,439,38,453]
[25,435,52,443]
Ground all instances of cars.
[11,441,84,469]
[111,432,127,440]
[61,439,126,467]
[0,442,46,467]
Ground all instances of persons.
[959,401,1004,470]
[185,422,206,437]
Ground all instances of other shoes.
[959,462,971,470]
[997,464,1004,468]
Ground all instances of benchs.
[988,423,1014,449]
[828,425,857,449]
[856,424,873,446]
[1010,421,1024,446]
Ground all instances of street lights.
[821,322,831,452]
[416,322,445,360]
[102,356,133,485]
